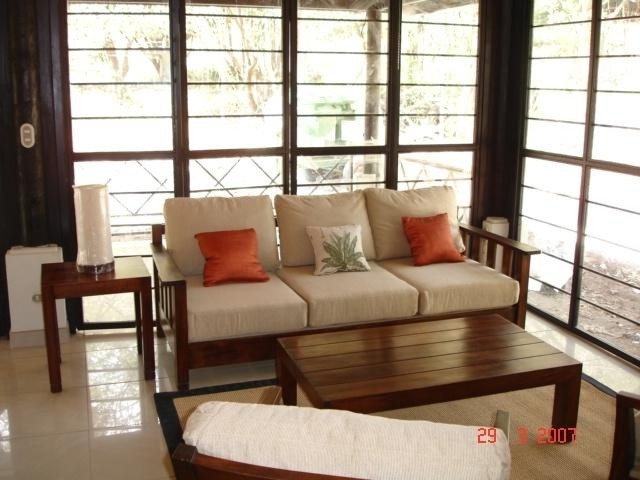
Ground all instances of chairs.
[609,390,640,480]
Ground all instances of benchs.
[171,401,510,479]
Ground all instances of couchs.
[150,185,541,391]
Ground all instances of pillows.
[164,194,279,277]
[401,213,465,266]
[193,228,270,288]
[305,224,371,276]
[274,189,376,268]
[364,186,465,262]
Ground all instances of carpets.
[154,372,616,480]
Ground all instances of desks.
[41,256,155,393]
